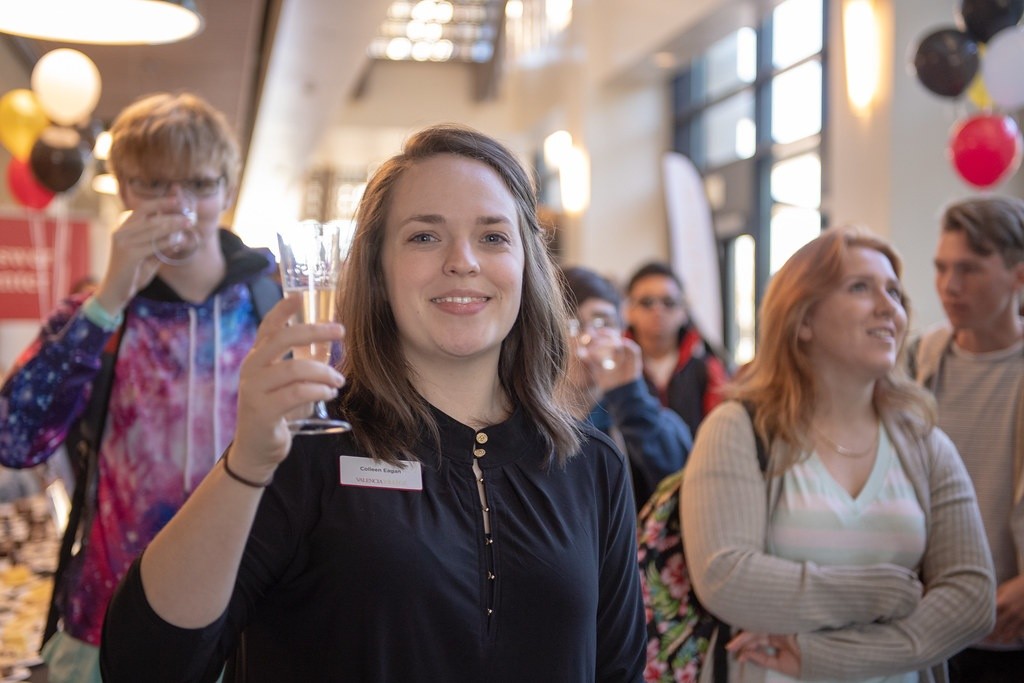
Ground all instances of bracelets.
[80,296,123,332]
[223,452,273,488]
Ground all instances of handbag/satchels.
[631,397,768,683]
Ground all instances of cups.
[564,317,625,370]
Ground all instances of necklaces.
[812,423,878,457]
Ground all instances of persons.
[0,93,344,683]
[901,196,1024,683]
[552,265,694,516]
[100,128,648,683]
[681,225,998,683]
[621,262,732,441]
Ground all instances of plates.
[0,560,48,683]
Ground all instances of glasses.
[117,172,225,199]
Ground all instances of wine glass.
[275,222,352,437]
[151,187,200,266]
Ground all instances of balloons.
[8,156,55,209]
[914,30,980,97]
[950,107,1019,190]
[30,138,85,192]
[961,0,1024,46]
[32,48,101,127]
[0,88,48,164]
